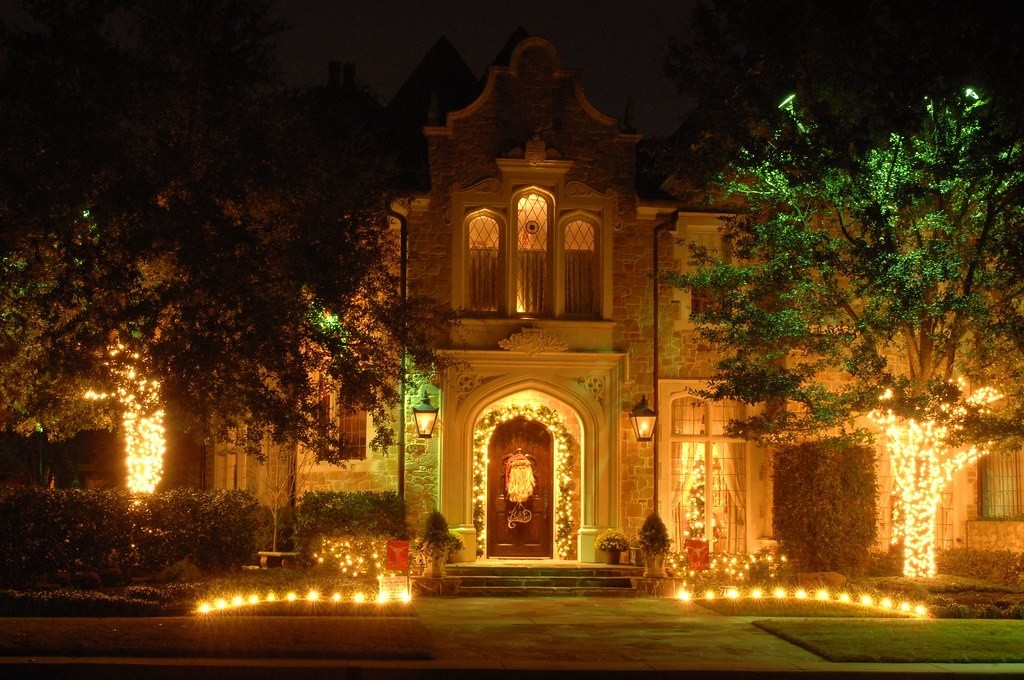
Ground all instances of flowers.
[636,513,673,558]
[412,512,464,556]
[447,527,464,553]
[595,529,630,551]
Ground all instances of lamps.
[628,394,659,442]
[414,391,439,439]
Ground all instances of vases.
[643,553,668,578]
[421,553,449,577]
[605,550,621,564]
